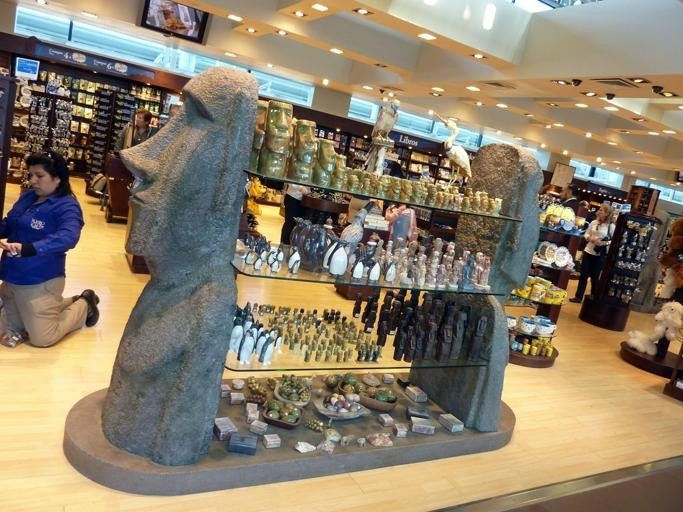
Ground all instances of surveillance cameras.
[652,86,663,93]
[572,79,582,86]
[606,93,615,99]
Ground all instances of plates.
[312,395,369,422]
[546,202,576,232]
[275,382,309,408]
[539,240,570,268]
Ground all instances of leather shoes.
[73,290,99,326]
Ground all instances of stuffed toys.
[626,216,683,365]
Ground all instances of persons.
[356,289,487,363]
[254,99,502,215]
[113,109,157,162]
[544,183,617,302]
[1,151,100,348]
[280,182,311,245]
[384,160,418,254]
[407,145,543,433]
[100,65,261,467]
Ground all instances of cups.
[511,337,553,357]
[505,312,557,335]
[519,275,567,305]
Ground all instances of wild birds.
[431,111,472,191]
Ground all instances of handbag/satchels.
[594,235,610,255]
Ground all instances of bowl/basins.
[338,379,349,395]
[359,388,399,412]
[263,401,305,429]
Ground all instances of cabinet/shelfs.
[0,51,182,222]
[61,64,545,497]
[506,178,660,368]
[292,104,469,186]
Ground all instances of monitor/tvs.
[136,0,213,46]
[677,171,683,182]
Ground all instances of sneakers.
[569,298,581,303]
